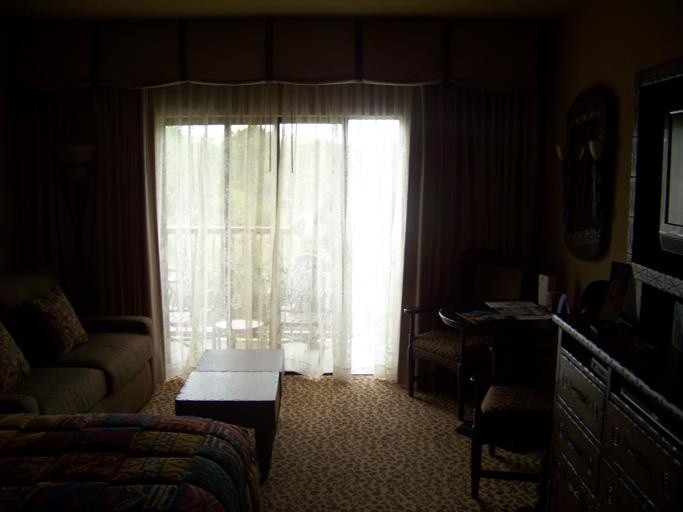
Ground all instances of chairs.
[403,299,558,500]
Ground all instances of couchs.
[0,265,160,414]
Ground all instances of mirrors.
[561,80,617,259]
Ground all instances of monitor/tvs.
[659,105,683,255]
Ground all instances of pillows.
[2,285,89,390]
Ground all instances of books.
[484,300,554,321]
[455,308,504,324]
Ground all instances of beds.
[1,413,263,512]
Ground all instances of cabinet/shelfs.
[550,63,683,512]
